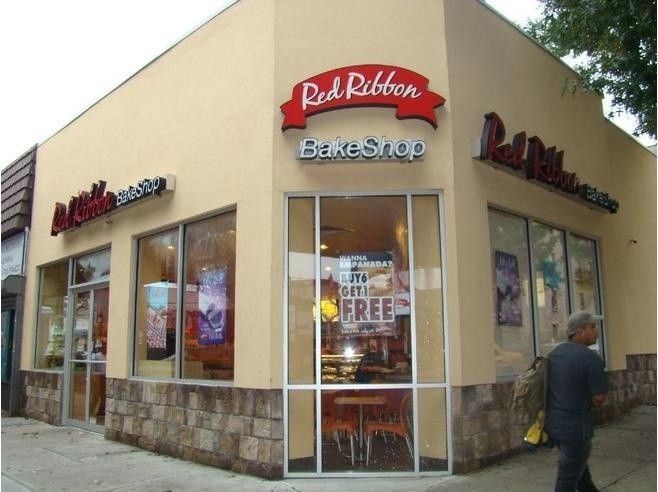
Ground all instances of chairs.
[320,387,414,469]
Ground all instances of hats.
[566,310,604,336]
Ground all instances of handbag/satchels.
[507,356,547,414]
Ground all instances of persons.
[541,310,611,491]
[354,349,396,385]
